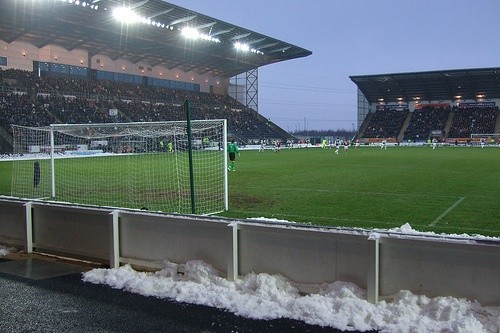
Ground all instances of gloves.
[238,152,241,159]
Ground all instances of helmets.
[231,139,234,143]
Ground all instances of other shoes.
[232,166,237,172]
[228,166,232,172]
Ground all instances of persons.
[0,67,311,157]
[227,139,240,172]
[335,137,340,154]
[321,137,349,151]
[361,105,500,149]
[355,137,360,150]
[381,139,388,151]
[432,137,438,149]
[479,135,486,148]
